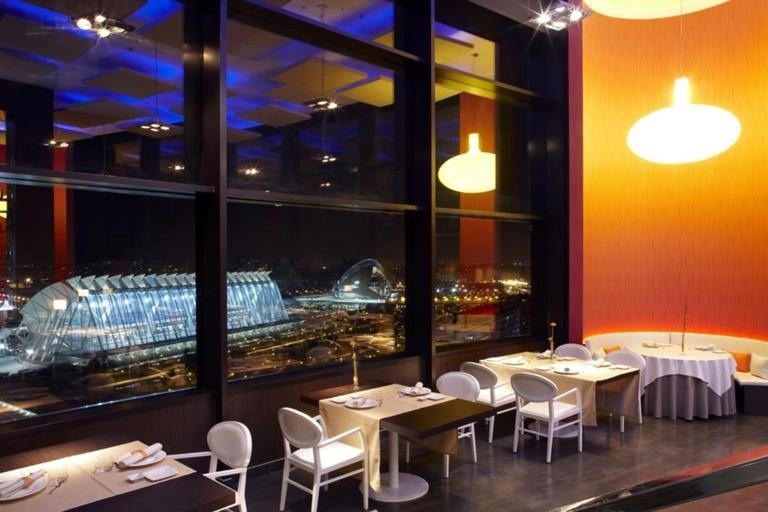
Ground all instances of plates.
[504,350,628,379]
[1,471,51,502]
[331,397,347,403]
[143,464,178,481]
[340,396,380,410]
[428,392,445,401]
[126,471,144,482]
[646,338,721,358]
[395,381,431,398]
[115,441,167,470]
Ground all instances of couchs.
[585,324,767,414]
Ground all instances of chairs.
[554,343,592,359]
[507,367,583,464]
[404,372,482,478]
[457,360,516,442]
[603,353,646,433]
[167,418,251,511]
[275,404,369,510]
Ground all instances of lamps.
[583,2,740,164]
[439,86,497,195]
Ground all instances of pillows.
[749,354,767,378]
[730,349,751,372]
[600,342,621,351]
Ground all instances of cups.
[96,453,113,474]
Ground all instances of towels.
[118,442,162,470]
[1,468,46,498]
[539,349,551,359]
[353,397,367,405]
[412,382,422,395]
